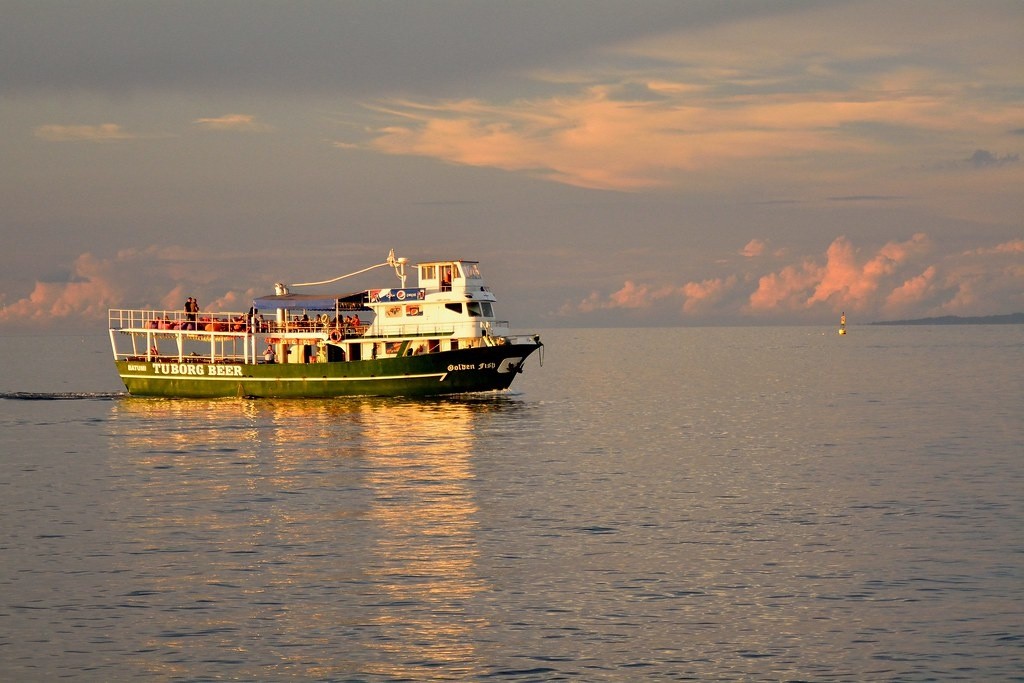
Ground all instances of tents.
[252,291,373,331]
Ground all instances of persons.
[144,345,160,363]
[262,344,275,364]
[143,298,361,332]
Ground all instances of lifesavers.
[321,314,331,326]
[330,330,342,343]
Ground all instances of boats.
[105,245,545,408]
